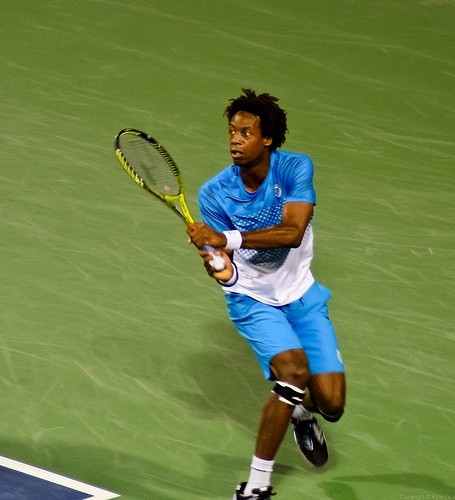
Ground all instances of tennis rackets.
[114,128,227,273]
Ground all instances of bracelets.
[218,261,239,288]
[221,229,243,251]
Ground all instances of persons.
[187,87,347,500]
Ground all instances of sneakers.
[232,482,277,500]
[290,414,328,467]
